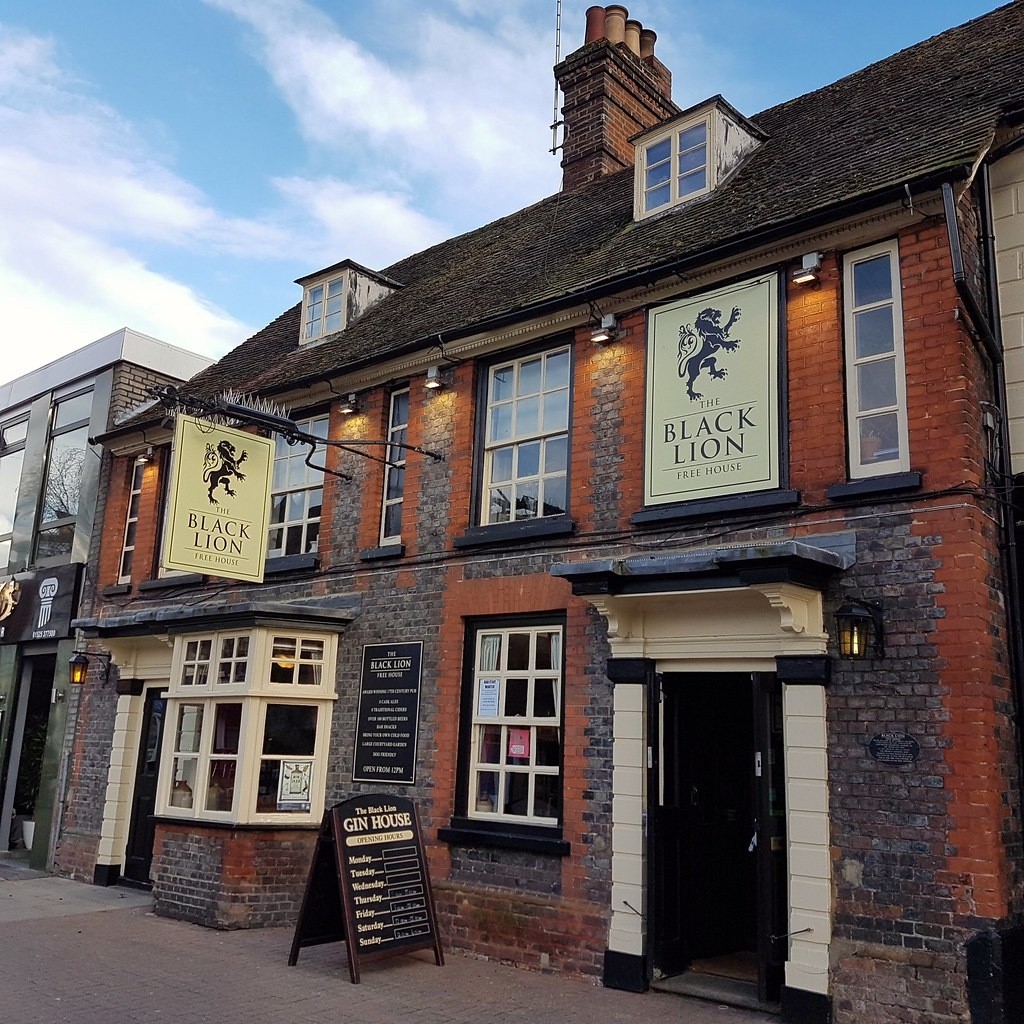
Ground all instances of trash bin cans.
[963,906,1024,1024]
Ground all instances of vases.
[860,437,880,459]
[23,819,38,851]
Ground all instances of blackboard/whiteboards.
[293,791,441,965]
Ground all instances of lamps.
[590,328,618,345]
[423,376,452,389]
[138,453,155,465]
[337,403,362,416]
[792,267,821,286]
[833,595,886,664]
[67,650,112,688]
